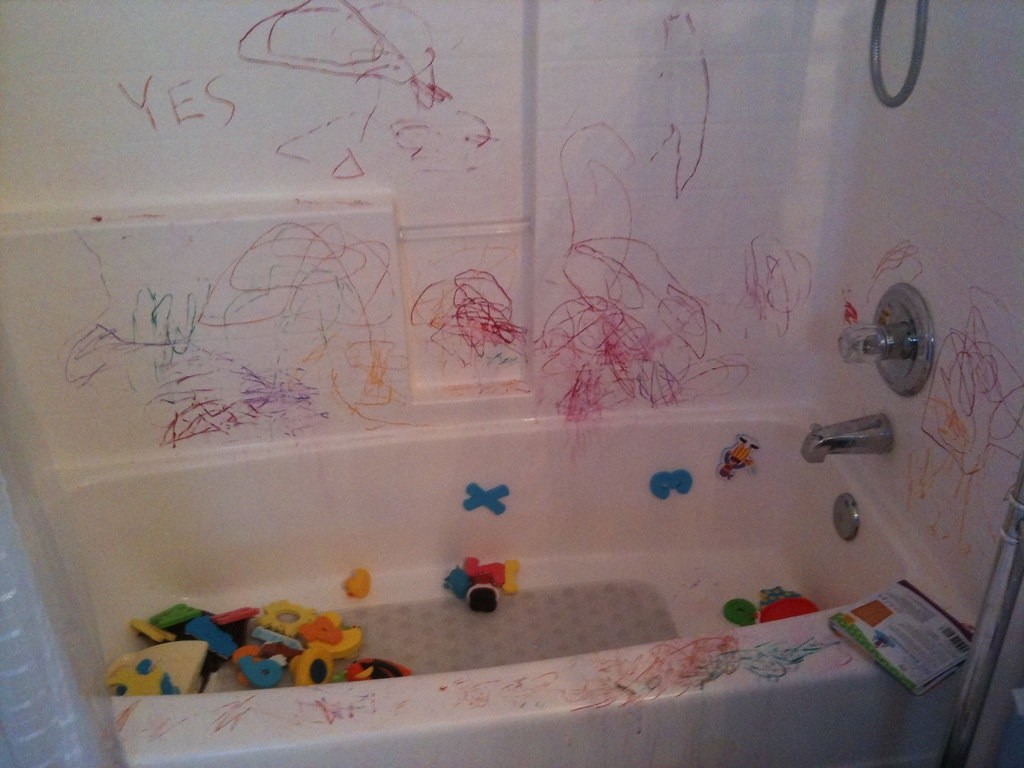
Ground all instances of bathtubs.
[35,402,977,767]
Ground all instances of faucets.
[799,414,893,464]
